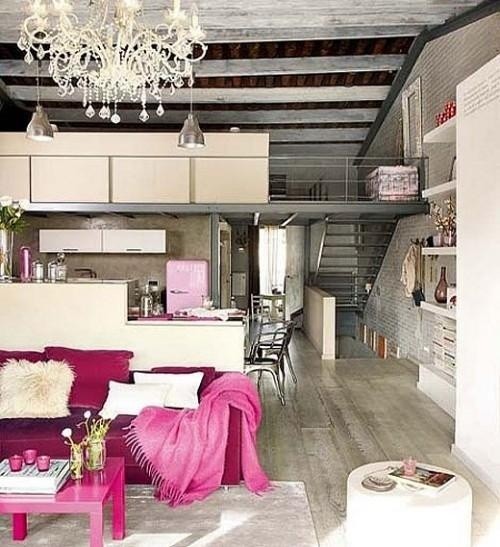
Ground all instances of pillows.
[0,346,216,419]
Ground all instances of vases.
[69,448,83,480]
[83,439,107,471]
[0,230,15,283]
[444,231,456,246]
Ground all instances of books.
[388,461,456,492]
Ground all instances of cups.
[23,450,37,465]
[10,455,23,472]
[403,456,417,476]
[37,455,50,471]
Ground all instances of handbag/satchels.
[412,288,425,306]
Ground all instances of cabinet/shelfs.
[416,114,457,420]
[0,132,270,205]
[39,229,167,254]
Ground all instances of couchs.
[0,370,243,485]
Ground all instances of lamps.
[16,0,210,149]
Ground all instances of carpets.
[0,480,320,547]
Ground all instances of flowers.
[61,428,85,476]
[428,199,456,231]
[75,410,112,467]
[0,196,31,236]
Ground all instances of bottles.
[140,284,153,318]
[231,295,237,308]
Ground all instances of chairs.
[244,294,297,406]
